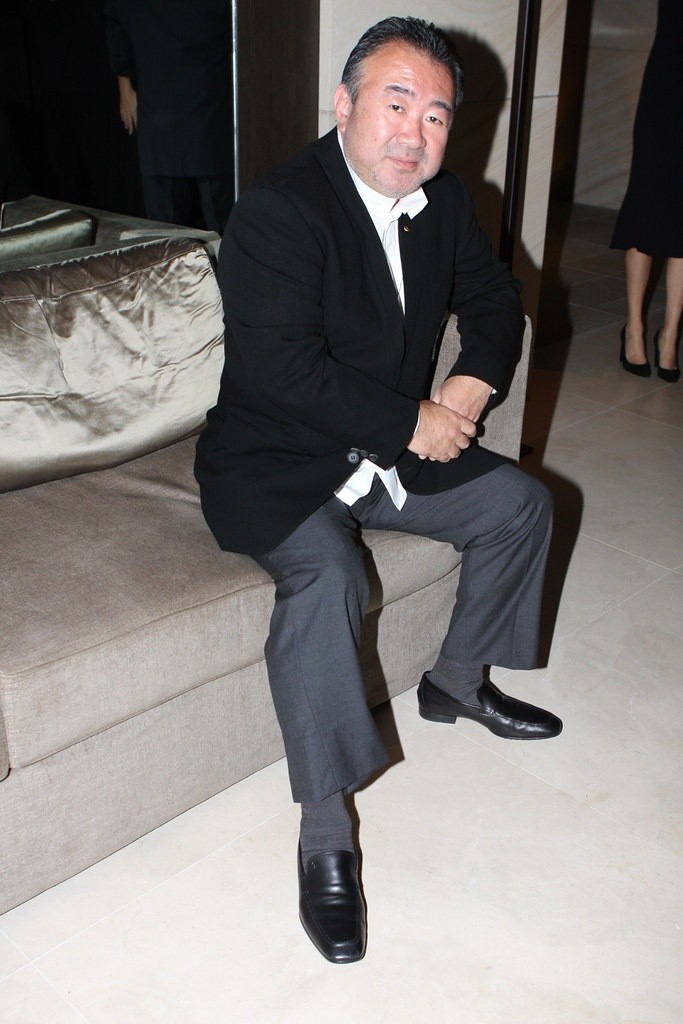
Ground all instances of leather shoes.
[298,826,367,965]
[418,670,566,740]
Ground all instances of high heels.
[620,323,651,378]
[653,327,680,384]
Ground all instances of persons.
[611,0,683,382]
[193,17,562,961]
[104,0,233,233]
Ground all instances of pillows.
[0,235,226,494]
[0,207,95,256]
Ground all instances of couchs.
[0,194,222,275]
[0,313,534,916]
